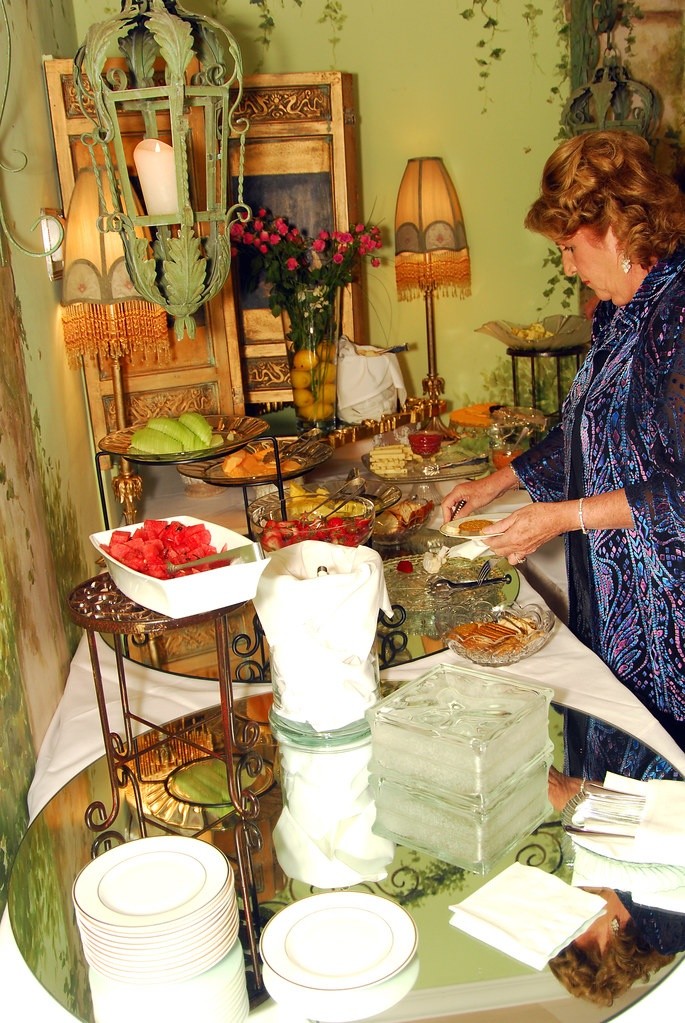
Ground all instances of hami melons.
[449,403,498,426]
[224,446,300,478]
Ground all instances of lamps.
[73,0,249,339]
[62,164,170,566]
[394,157,473,447]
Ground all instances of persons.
[439,129,685,721]
[548,700,685,1007]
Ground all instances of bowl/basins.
[249,494,375,549]
[435,600,555,667]
[88,514,271,621]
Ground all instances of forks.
[476,556,503,586]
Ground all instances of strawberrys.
[397,561,413,573]
[261,513,371,550]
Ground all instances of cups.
[408,430,443,460]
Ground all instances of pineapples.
[287,480,369,520]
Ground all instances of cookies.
[459,520,493,531]
[447,623,523,660]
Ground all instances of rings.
[514,553,526,564]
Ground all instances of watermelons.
[99,519,232,580]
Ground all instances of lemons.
[288,340,336,419]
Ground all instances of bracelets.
[579,498,590,535]
[580,777,589,796]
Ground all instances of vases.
[281,285,343,432]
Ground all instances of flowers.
[230,205,392,409]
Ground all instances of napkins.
[450,539,497,561]
[448,771,685,971]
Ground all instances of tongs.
[298,477,367,530]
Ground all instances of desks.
[0,432,685,1022]
[507,345,590,451]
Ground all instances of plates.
[262,953,420,1023]
[98,414,271,466]
[71,835,250,987]
[258,888,418,991]
[246,480,402,517]
[474,314,592,351]
[89,938,250,1023]
[360,445,491,484]
[373,553,502,636]
[560,788,685,872]
[177,440,335,488]
[439,512,512,540]
